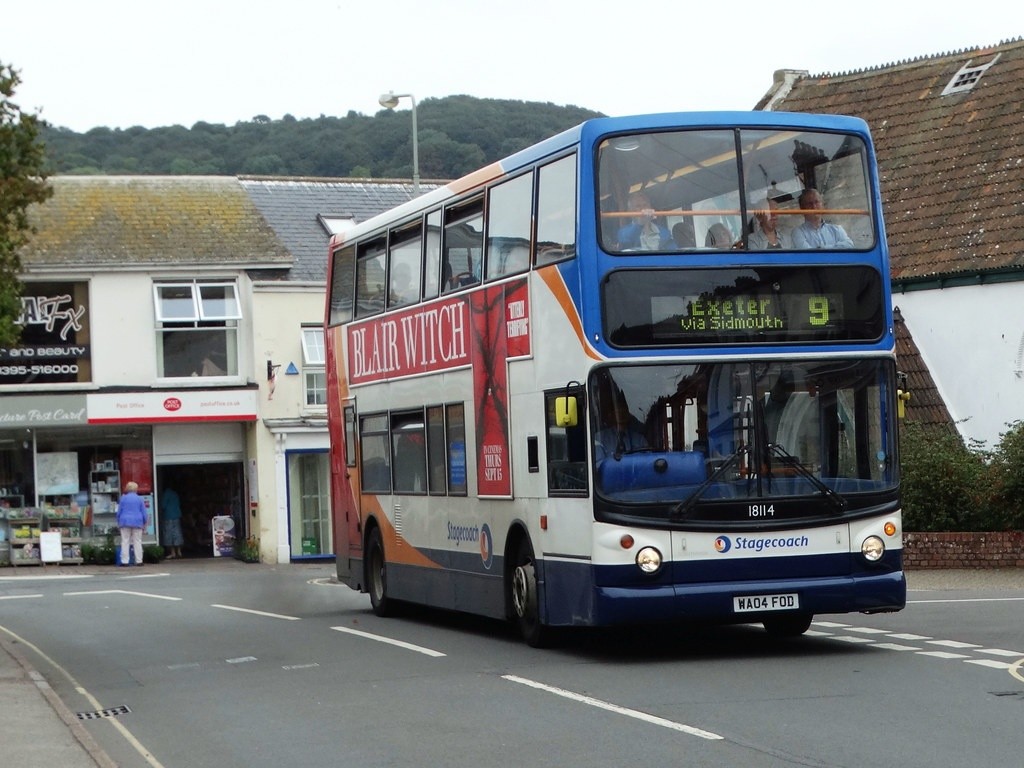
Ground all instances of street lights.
[378,92,422,200]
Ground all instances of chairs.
[446,271,480,294]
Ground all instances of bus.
[323,110,912,650]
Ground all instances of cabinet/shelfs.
[0,469,120,569]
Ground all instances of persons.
[672,221,695,248]
[705,223,733,248]
[600,181,632,244]
[158,476,185,560]
[747,199,796,250]
[791,189,856,250]
[594,396,651,471]
[117,482,148,566]
[617,191,679,250]
